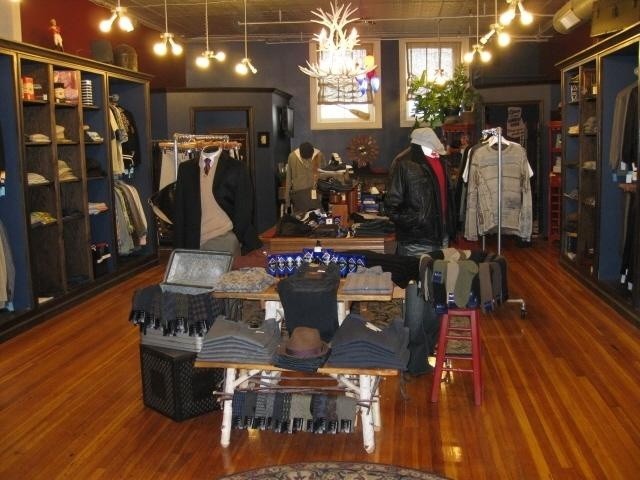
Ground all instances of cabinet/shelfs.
[0,37,159,344]
[547,121,562,241]
[554,21,640,328]
[259,223,398,256]
[193,277,407,455]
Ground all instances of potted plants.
[405,61,483,140]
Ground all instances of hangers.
[480,127,522,148]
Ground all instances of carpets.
[216,461,452,480]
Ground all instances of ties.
[204,158,211,176]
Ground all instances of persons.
[175,145,263,257]
[49,17,64,52]
[284,142,327,213]
[384,127,455,377]
[344,147,358,173]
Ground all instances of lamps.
[432,0,534,86]
[195,0,226,69]
[154,0,183,57]
[297,0,382,97]
[98,0,135,33]
[234,0,257,76]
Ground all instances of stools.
[431,308,484,406]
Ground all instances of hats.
[277,327,329,359]
[409,127,447,156]
